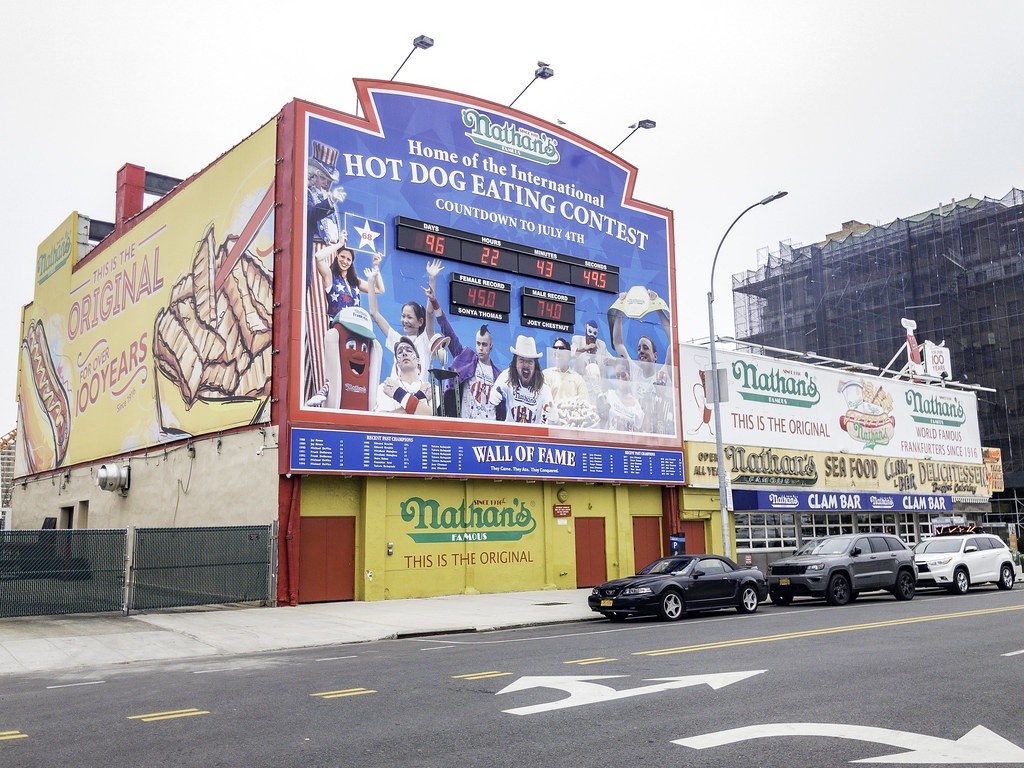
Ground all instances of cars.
[588,554,769,623]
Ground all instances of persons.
[541,338,594,427]
[569,321,622,393]
[363,257,445,379]
[420,282,507,421]
[611,289,675,434]
[313,229,386,331]
[593,359,652,433]
[489,335,558,425]
[308,140,347,402]
[375,335,443,416]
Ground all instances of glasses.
[615,371,629,379]
[553,345,568,350]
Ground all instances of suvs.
[763,533,919,608]
[904,530,1017,596]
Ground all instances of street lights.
[705,190,790,554]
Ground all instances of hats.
[331,307,377,340]
[587,320,598,328]
[428,332,450,360]
[308,140,340,181]
[509,335,543,359]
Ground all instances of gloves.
[488,386,507,407]
[330,186,347,203]
[542,401,559,425]
